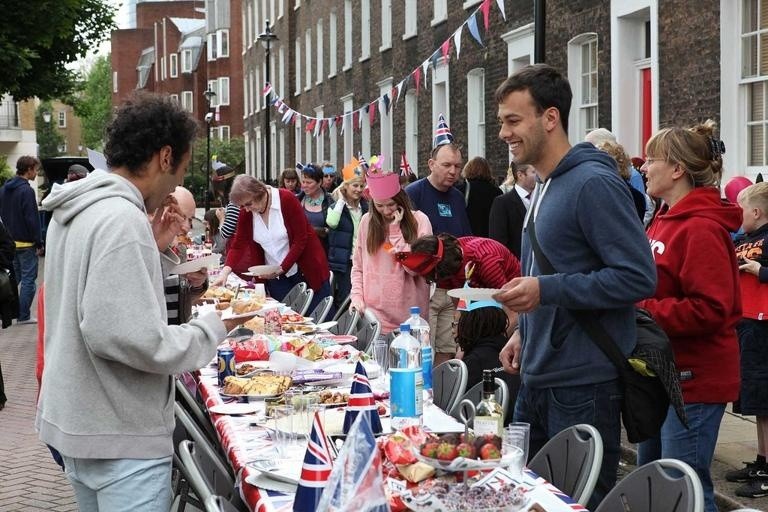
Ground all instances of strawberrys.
[421,432,502,460]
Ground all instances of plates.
[447,287,500,302]
[207,402,262,416]
[234,361,274,378]
[264,414,399,439]
[169,254,339,333]
[413,441,524,471]
[323,362,380,380]
[399,483,537,512]
[252,456,300,484]
[316,335,359,344]
[315,386,354,407]
[218,386,281,399]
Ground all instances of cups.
[503,422,532,479]
[262,310,283,352]
[273,390,327,453]
[370,339,388,387]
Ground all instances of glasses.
[239,200,251,209]
[645,156,677,166]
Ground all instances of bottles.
[388,324,424,428]
[203,228,214,254]
[403,307,434,412]
[475,367,504,438]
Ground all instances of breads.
[223,373,293,395]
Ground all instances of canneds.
[217,348,235,387]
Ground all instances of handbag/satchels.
[616,306,688,442]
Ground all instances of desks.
[194,276,594,512]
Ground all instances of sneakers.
[726,460,767,498]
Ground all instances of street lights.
[42,108,52,160]
[77,141,83,157]
[257,19,280,186]
[202,84,216,213]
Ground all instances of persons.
[36,94,256,511]
[36,164,196,473]
[494,62,661,512]
[1,156,45,328]
[205,140,537,427]
[586,119,768,512]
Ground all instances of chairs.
[172,276,707,512]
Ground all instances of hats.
[396,150,415,177]
[433,112,456,148]
